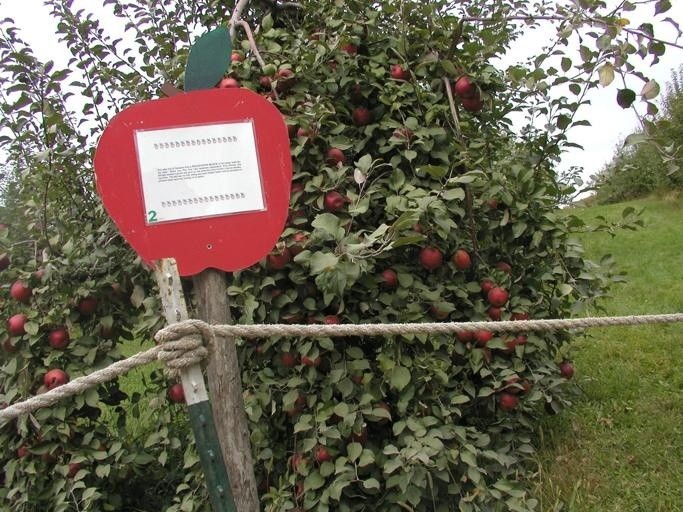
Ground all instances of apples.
[2,43,574,470]
